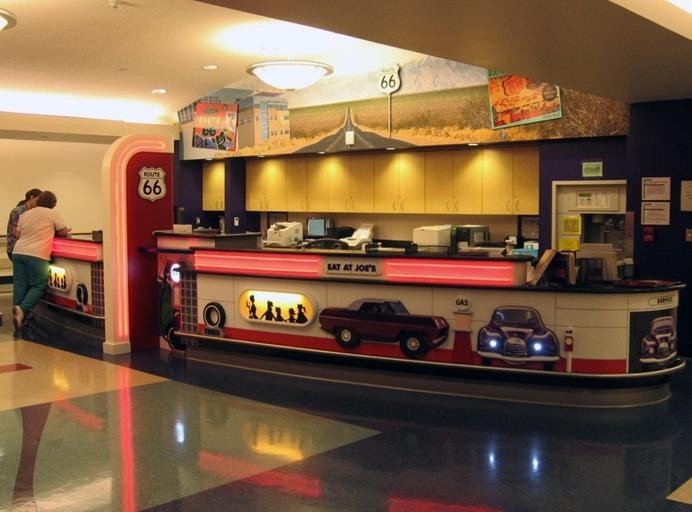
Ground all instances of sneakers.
[13,305,35,330]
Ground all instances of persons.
[12,191,69,329]
[7,189,42,261]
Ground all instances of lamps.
[0,9,17,32]
[247,60,335,91]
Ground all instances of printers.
[339,223,374,250]
[413,224,452,247]
[266,221,303,245]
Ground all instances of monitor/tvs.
[306,216,328,239]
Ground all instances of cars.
[642,316,682,366]
[476,303,561,367]
[317,297,449,360]
